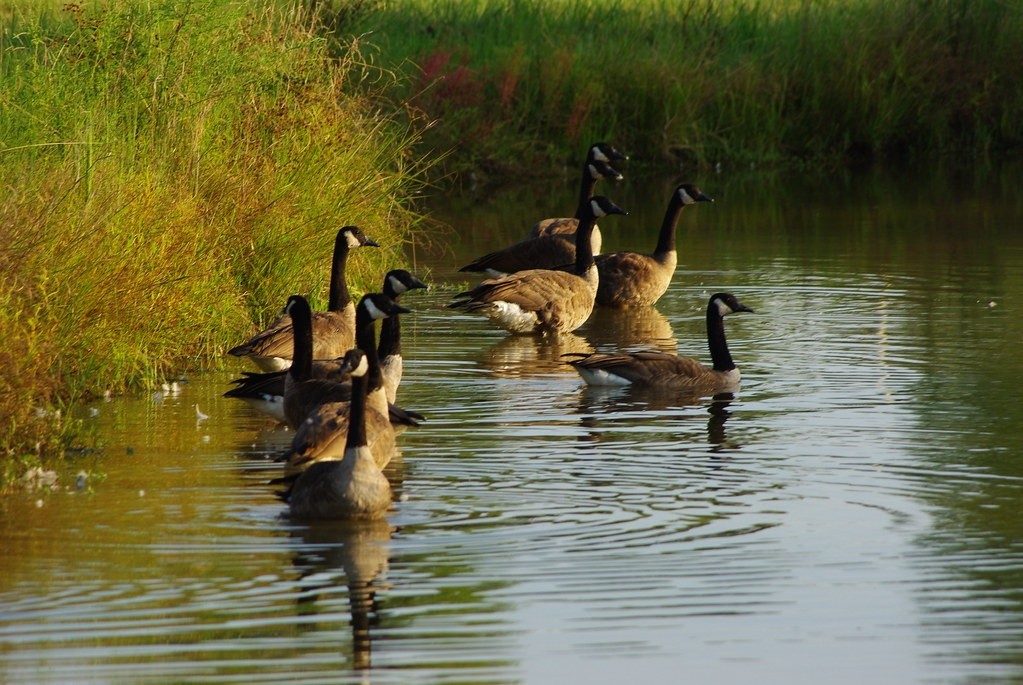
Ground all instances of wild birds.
[444,143,756,387]
[221,225,428,517]
